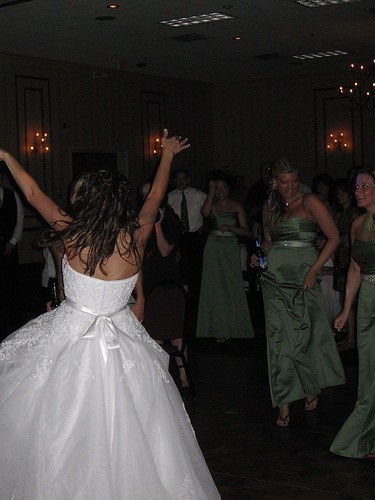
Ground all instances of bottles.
[254,237,268,271]
[47,277,62,311]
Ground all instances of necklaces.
[283,199,296,206]
[364,216,374,230]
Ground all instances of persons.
[329,168,375,458]
[0,128,222,500]
[42,168,359,392]
[0,167,25,329]
[250,158,346,429]
[196,175,255,344]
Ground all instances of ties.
[181,192,190,234]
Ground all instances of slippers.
[305,393,320,411]
[277,408,292,428]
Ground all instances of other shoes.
[177,366,190,395]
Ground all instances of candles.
[327,131,348,153]
[30,131,49,154]
[153,138,162,156]
[338,59,375,107]
